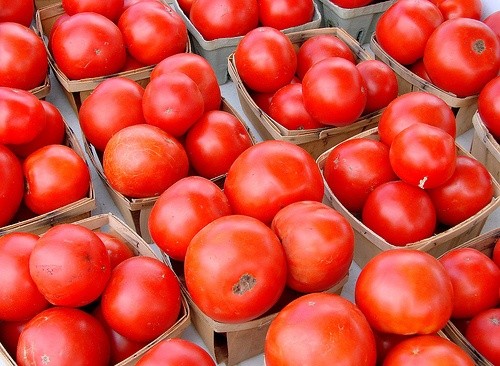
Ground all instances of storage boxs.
[0,0,500,366]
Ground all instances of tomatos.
[0,0,500,366]
